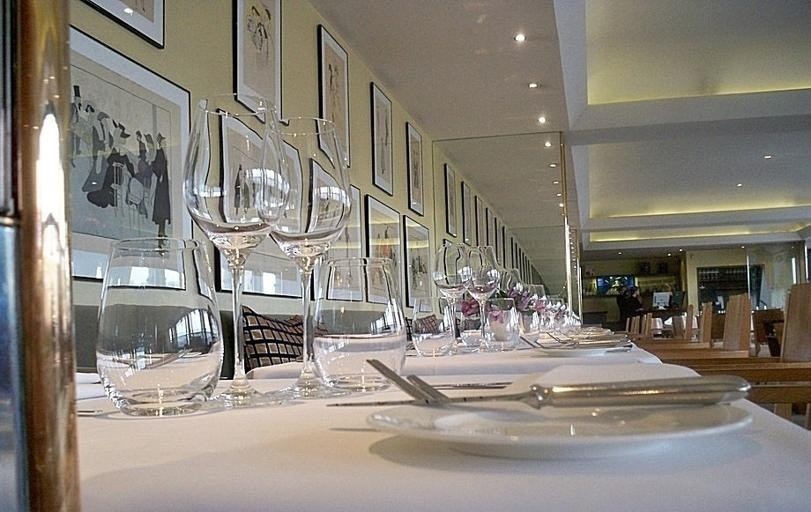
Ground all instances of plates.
[539,344,629,360]
[361,397,755,467]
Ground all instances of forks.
[516,327,634,351]
[364,355,753,404]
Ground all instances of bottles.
[696,267,747,281]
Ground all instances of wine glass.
[500,267,580,354]
[433,241,470,355]
[178,97,288,407]
[466,247,503,353]
[253,113,352,401]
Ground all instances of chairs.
[0,280,809,512]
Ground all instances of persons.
[235,163,242,216]
[617,285,645,330]
[242,170,251,217]
[71,85,172,257]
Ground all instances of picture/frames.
[443,239,460,285]
[402,214,433,308]
[404,120,424,217]
[443,161,458,239]
[214,106,306,299]
[230,1,284,127]
[314,22,353,171]
[67,21,194,295]
[364,193,403,307]
[79,0,166,50]
[369,80,394,197]
[307,156,365,304]
[461,178,551,315]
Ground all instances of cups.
[458,299,484,351]
[411,294,456,356]
[481,296,520,353]
[311,256,405,390]
[96,237,227,415]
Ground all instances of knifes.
[316,375,751,405]
